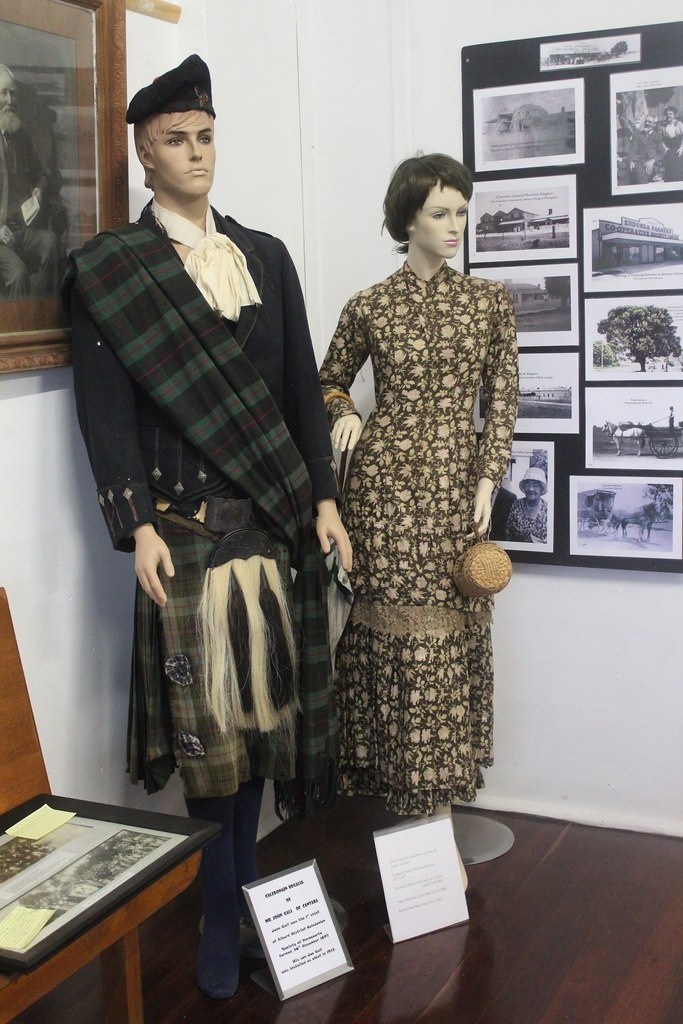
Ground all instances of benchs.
[0,585,204,1024]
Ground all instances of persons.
[657,105,682,181]
[487,479,517,541]
[669,406,676,429]
[64,52,358,1000]
[505,467,548,543]
[314,153,527,819]
[627,115,664,183]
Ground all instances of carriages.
[577,489,658,544]
[602,421,683,459]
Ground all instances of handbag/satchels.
[456,520,513,596]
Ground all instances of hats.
[519,467,548,496]
[126,53,216,123]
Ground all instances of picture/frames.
[460,20,683,575]
[242,859,355,1001]
[0,792,224,978]
[0,0,131,376]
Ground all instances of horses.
[616,506,661,541]
[601,421,647,457]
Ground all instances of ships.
[517,370,571,402]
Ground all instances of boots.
[184,787,242,1000]
[235,777,265,916]
